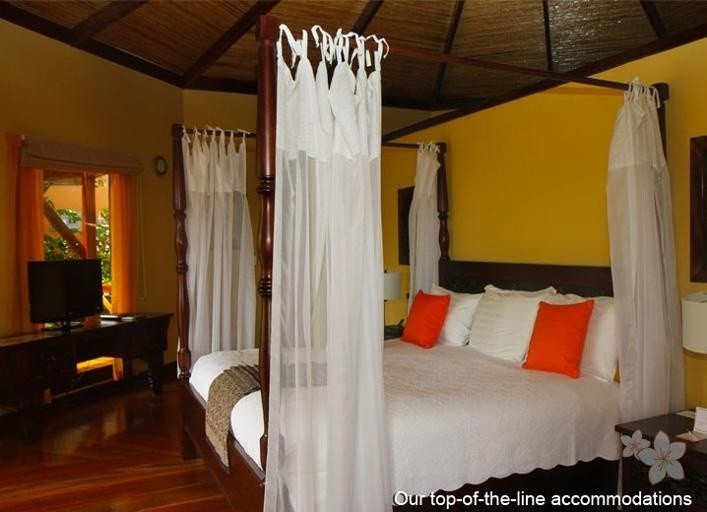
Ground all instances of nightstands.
[615,407,707,508]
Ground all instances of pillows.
[402,284,622,384]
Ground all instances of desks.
[0,310,173,439]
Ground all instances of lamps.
[683,289,707,435]
[383,267,403,337]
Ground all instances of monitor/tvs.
[27,259,102,331]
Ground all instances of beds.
[186,261,625,510]
